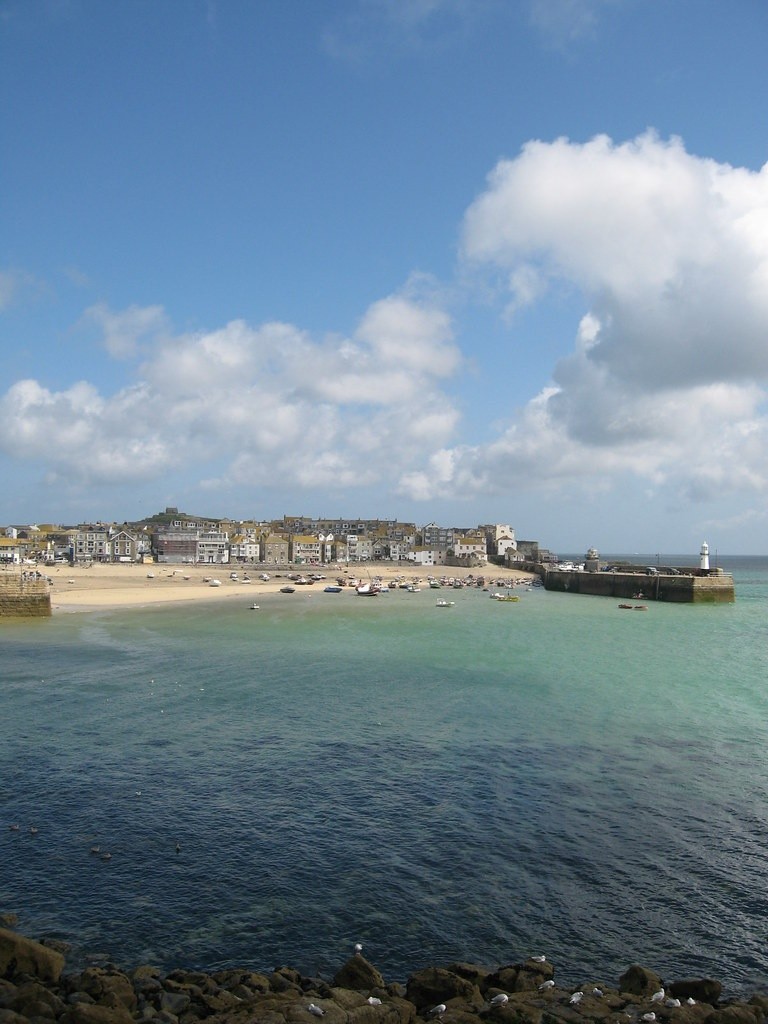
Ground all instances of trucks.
[119,556,135,563]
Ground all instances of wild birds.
[592,987,604,998]
[687,997,696,1006]
[664,998,681,1007]
[641,1012,655,1021]
[366,997,382,1005]
[568,992,583,1006]
[650,988,664,1006]
[427,1004,447,1019]
[531,955,546,963]
[355,944,363,955]
[488,994,509,1008]
[309,1004,328,1017]
[538,980,555,990]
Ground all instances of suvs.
[646,567,659,575]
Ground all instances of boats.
[250,603,260,610]
[230,572,270,584]
[208,579,220,586]
[274,571,534,596]
[635,604,650,611]
[183,576,190,580]
[497,596,519,602]
[618,603,634,609]
[436,598,455,607]
[203,576,210,583]
[147,571,154,578]
